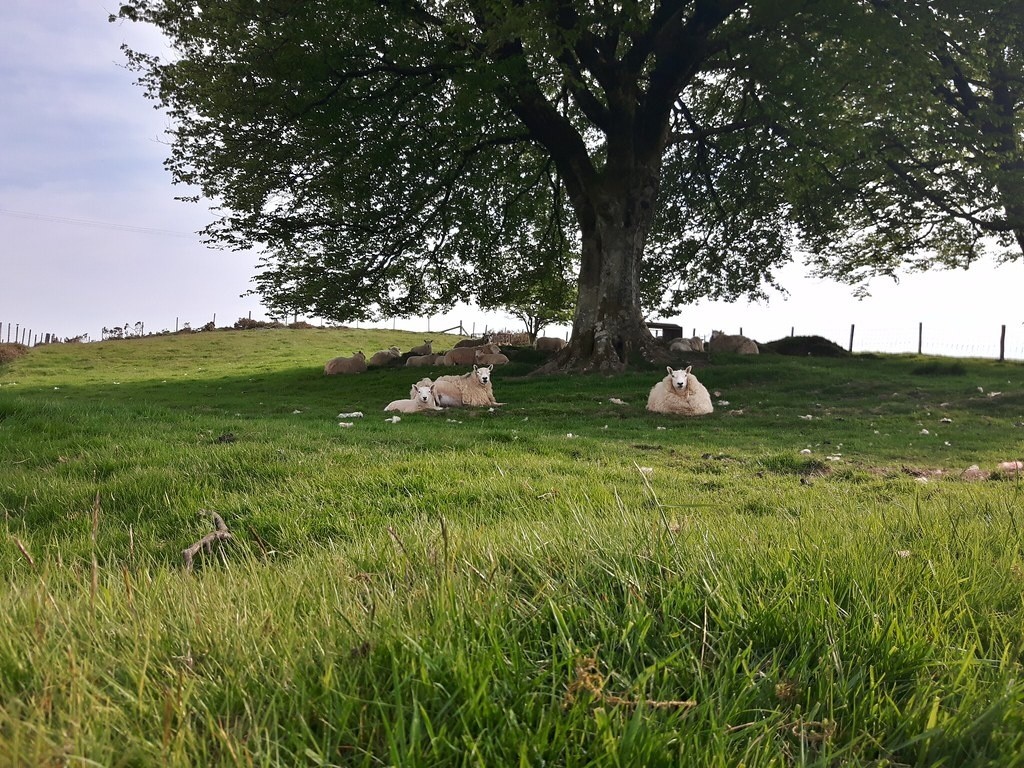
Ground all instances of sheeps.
[709,330,759,355]
[406,339,444,366]
[370,339,401,364]
[444,333,509,365]
[536,337,569,352]
[670,336,704,352]
[385,365,507,414]
[325,350,368,374]
[647,364,714,416]
